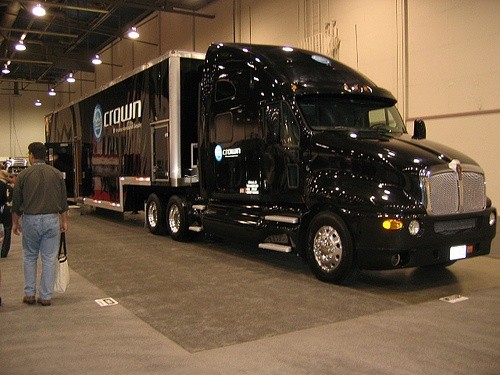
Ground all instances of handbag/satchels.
[54,231,70,292]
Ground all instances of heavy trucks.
[43,41,497,286]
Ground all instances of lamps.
[49,88,56,96]
[35,98,42,107]
[67,71,75,83]
[1,64,10,73]
[128,25,139,38]
[31,2,46,16]
[14,40,26,51]
[91,54,102,65]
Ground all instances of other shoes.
[23,295,36,303]
[38,297,51,305]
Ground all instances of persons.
[0,170,17,258]
[11,142,69,306]
